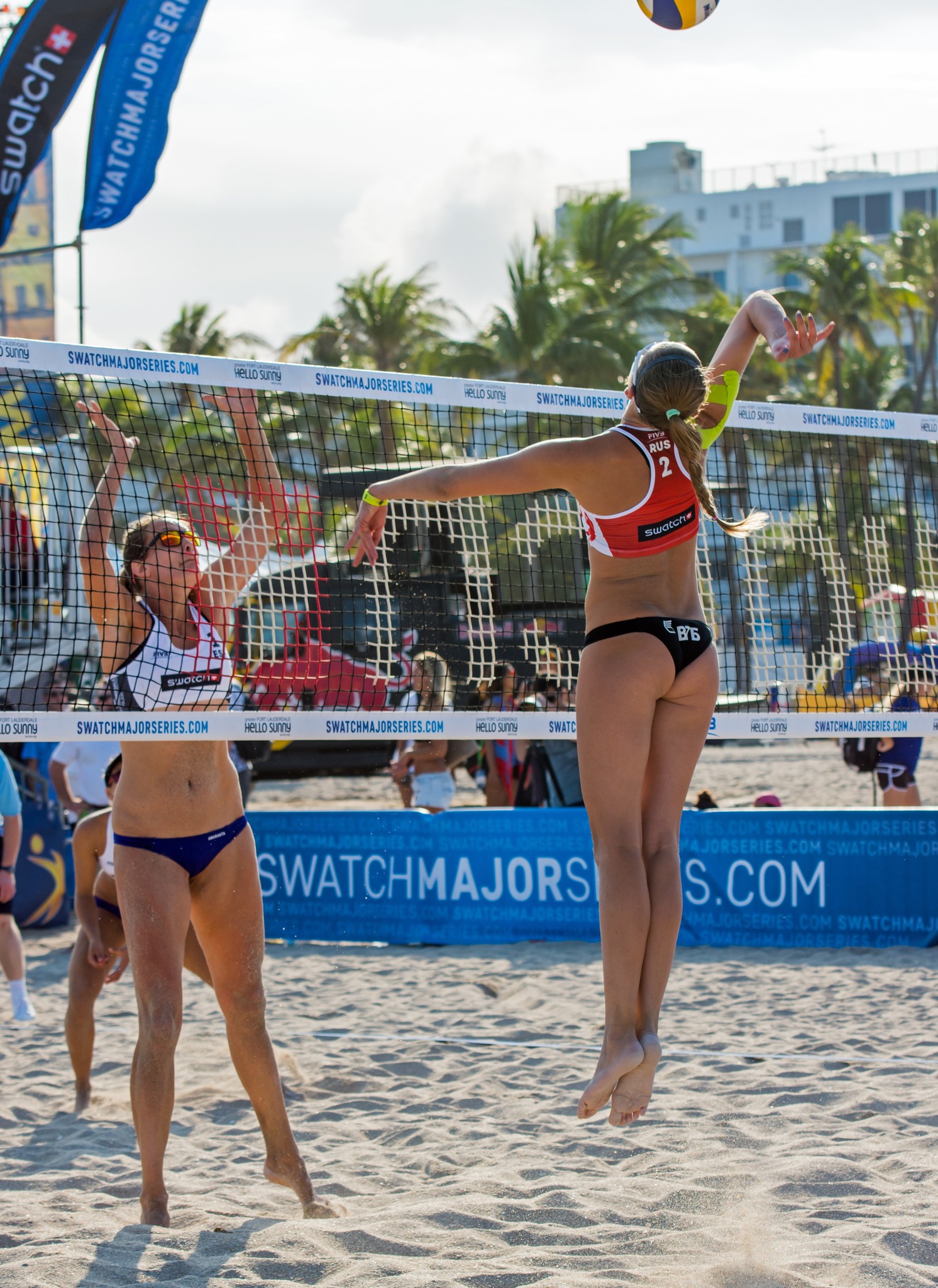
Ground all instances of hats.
[516,696,543,711]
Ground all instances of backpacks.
[843,704,880,773]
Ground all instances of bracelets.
[362,489,388,506]
[0,865,16,872]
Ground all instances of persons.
[75,381,316,1230]
[388,650,585,812]
[0,650,122,831]
[344,289,837,1128]
[62,755,218,1117]
[0,747,38,1021]
[842,653,923,806]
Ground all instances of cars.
[0,455,589,813]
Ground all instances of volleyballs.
[635,0,720,31]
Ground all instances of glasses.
[107,770,121,788]
[139,529,201,559]
[547,694,556,703]
[49,690,67,698]
[98,693,112,701]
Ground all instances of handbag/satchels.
[444,740,479,769]
